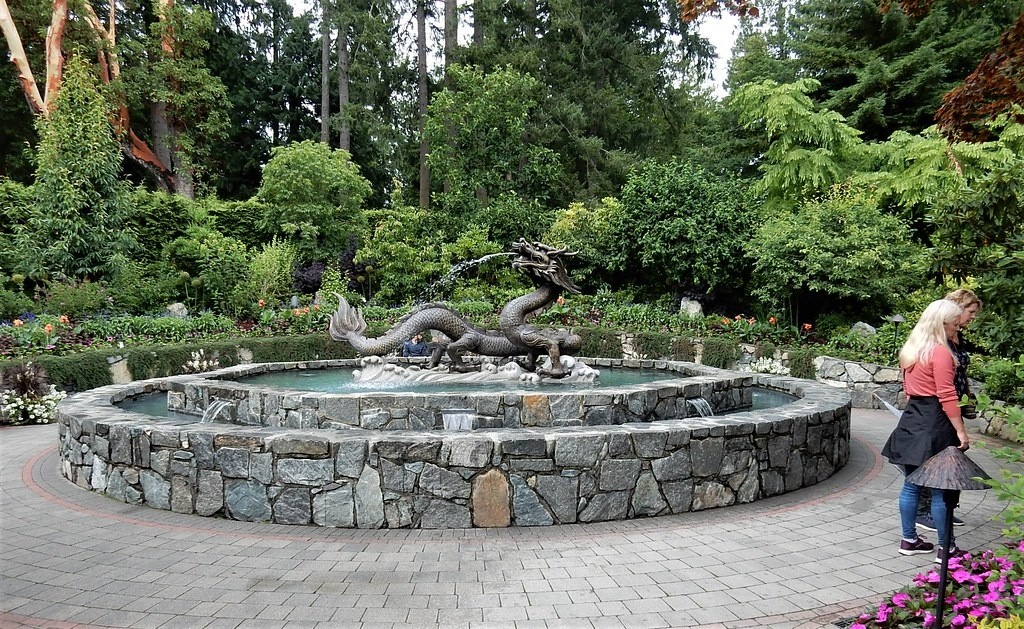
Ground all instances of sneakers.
[935,544,969,564]
[898,537,934,556]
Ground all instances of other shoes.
[916,513,938,532]
[953,516,964,526]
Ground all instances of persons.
[403,333,429,357]
[880,298,969,565]
[915,289,977,531]
